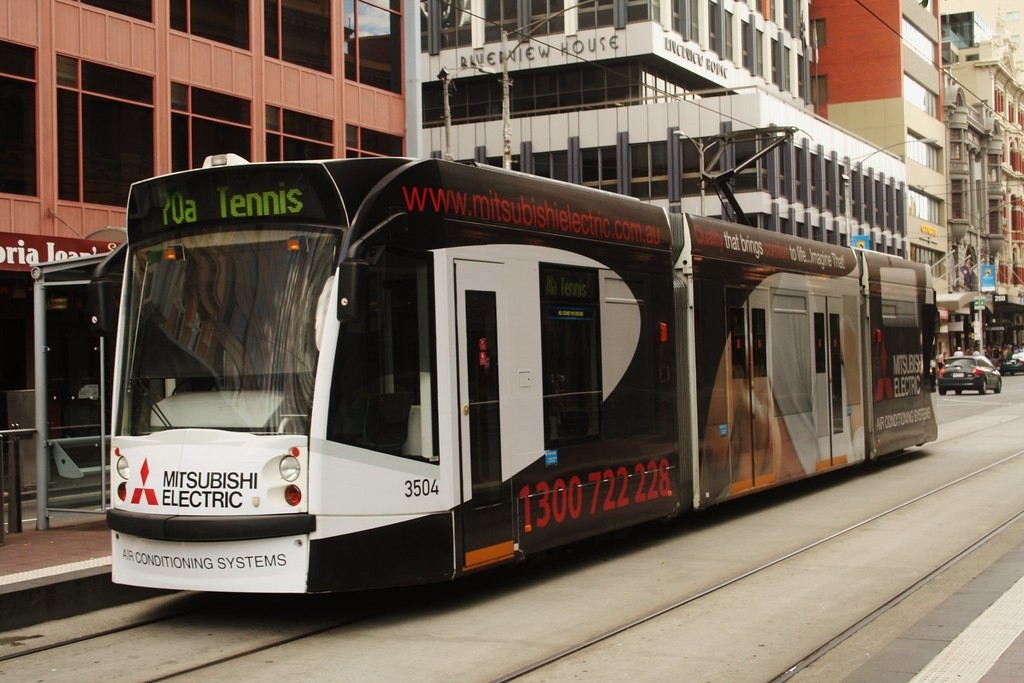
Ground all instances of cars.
[997,349,1024,376]
[938,355,1002,395]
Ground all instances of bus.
[106,127,938,594]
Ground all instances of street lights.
[842,139,937,246]
[672,130,718,218]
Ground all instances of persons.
[938,352,946,370]
[953,342,1024,370]
